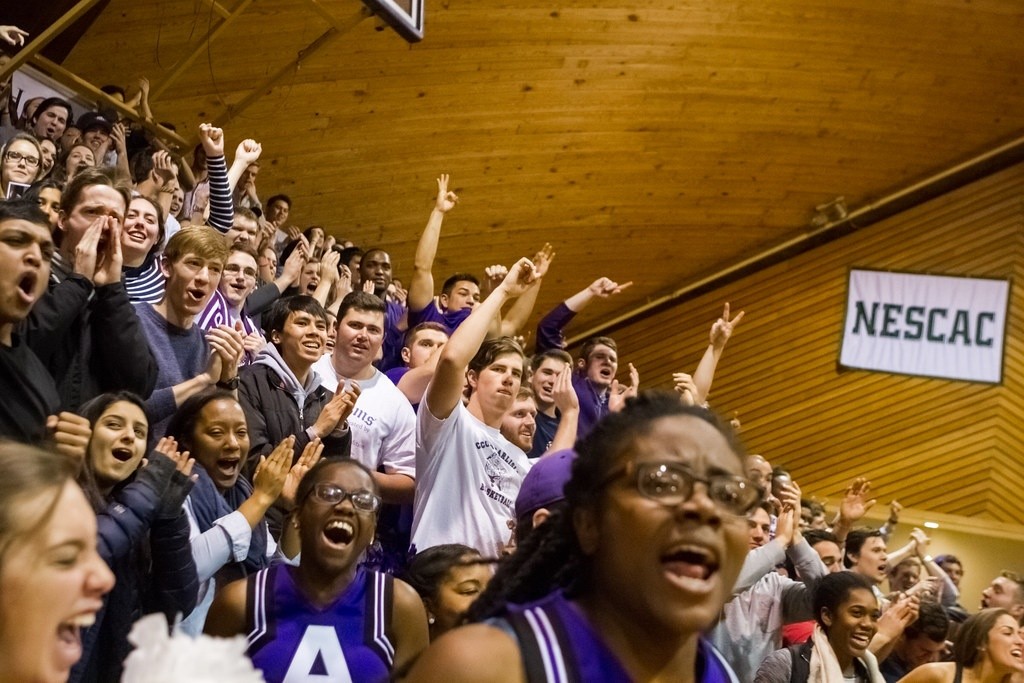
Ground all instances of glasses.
[223,263,258,280]
[604,452,767,521]
[2,151,43,168]
[869,528,887,536]
[309,481,386,516]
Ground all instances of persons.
[0,26,1024,683]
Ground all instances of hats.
[77,111,112,134]
[515,449,577,517]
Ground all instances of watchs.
[216,376,240,391]
[192,203,204,213]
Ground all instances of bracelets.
[158,184,173,193]
[904,590,910,597]
[923,554,936,564]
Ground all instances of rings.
[301,461,307,465]
[271,458,278,463]
